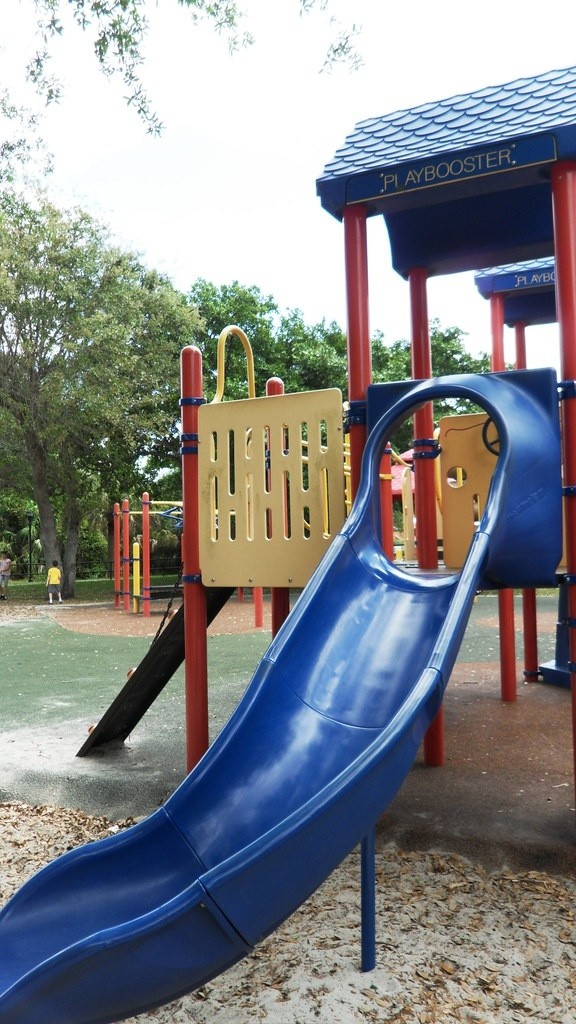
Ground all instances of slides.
[0,369,562,1024]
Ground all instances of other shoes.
[0,595,6,600]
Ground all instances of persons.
[45,560,62,603]
[0,551,11,599]
